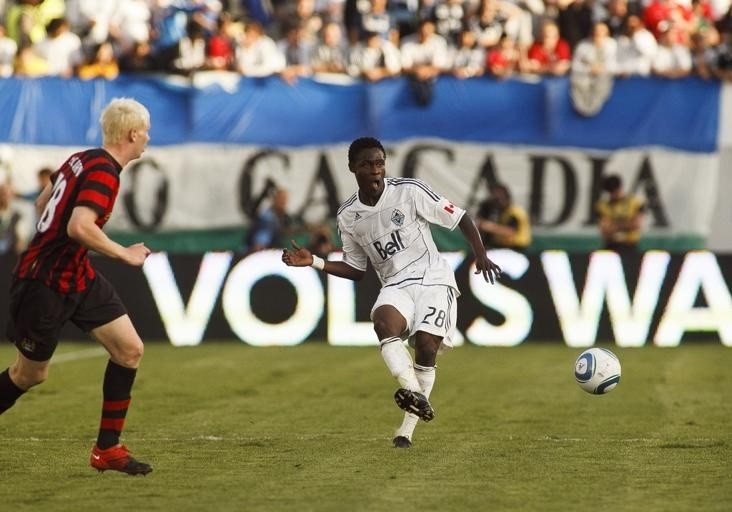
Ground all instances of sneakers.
[90,443,154,476]
[393,432,413,448]
[394,388,435,423]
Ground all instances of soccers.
[574,347,621,394]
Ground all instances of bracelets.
[309,254,327,271]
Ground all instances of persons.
[38,168,54,189]
[1,184,29,253]
[0,0,731,86]
[280,135,503,446]
[1,95,156,477]
[248,186,290,252]
[475,185,534,251]
[594,172,647,250]
[308,225,342,254]
